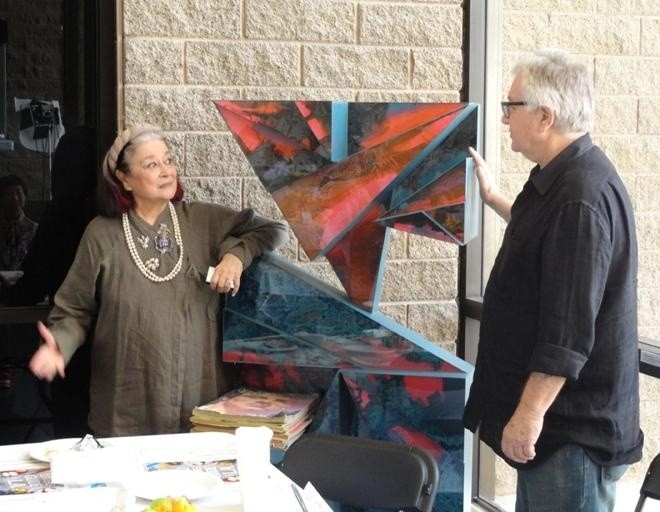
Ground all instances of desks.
[0,431,331,512]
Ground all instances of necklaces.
[121,201,184,282]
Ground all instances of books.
[189,387,321,451]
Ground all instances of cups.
[231,426,272,474]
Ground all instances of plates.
[29,438,108,464]
[130,469,217,502]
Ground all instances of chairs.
[278,430,437,512]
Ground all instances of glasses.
[501,100,527,118]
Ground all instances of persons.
[0,173,39,271]
[28,122,289,437]
[464,49,645,512]
